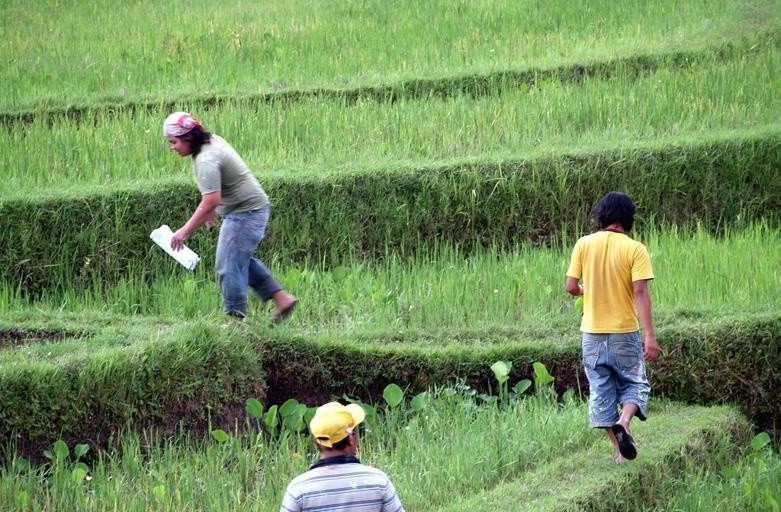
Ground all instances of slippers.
[268,298,299,329]
[611,423,638,460]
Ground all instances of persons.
[279,402,405,512]
[162,111,299,333]
[566,190,659,463]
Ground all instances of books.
[150,226,201,272]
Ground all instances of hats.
[309,400,366,448]
